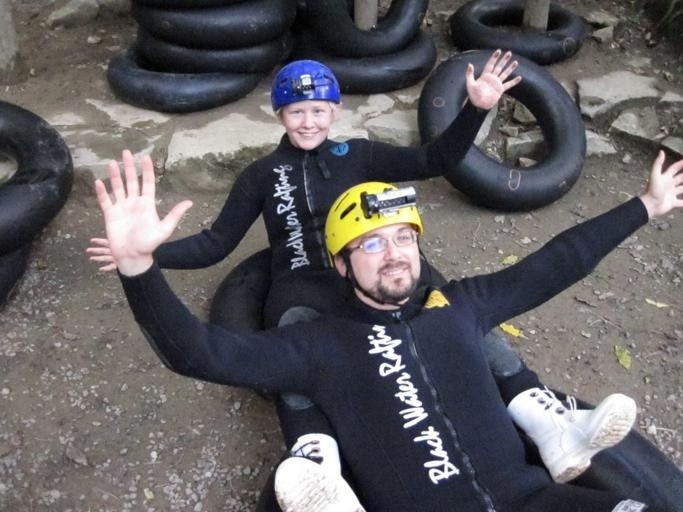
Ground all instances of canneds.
[251,388,683,512]
[209,246,447,406]
[0,239,36,313]
[0,99,74,259]
[418,47,585,211]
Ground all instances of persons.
[80,44,640,512]
[92,146,683,512]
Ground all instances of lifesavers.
[251,388,683,512]
[0,99,74,259]
[418,47,585,211]
[209,246,447,406]
[453,0,585,64]
[309,0,428,51]
[291,29,435,94]
[0,239,36,313]
[106,0,289,111]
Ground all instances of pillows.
[324,181,423,268]
[270,59,341,110]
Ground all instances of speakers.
[505,386,635,483]
[274,433,367,512]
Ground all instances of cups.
[349,230,419,253]
